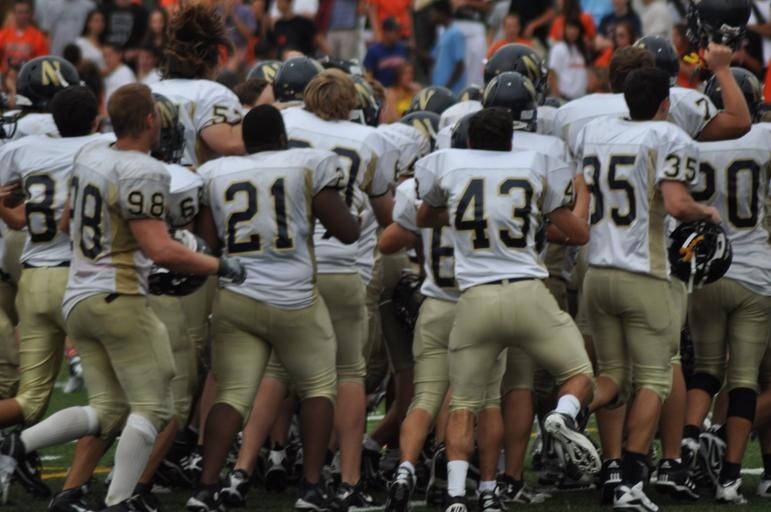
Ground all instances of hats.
[385,16,401,31]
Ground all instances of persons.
[0,0,771,512]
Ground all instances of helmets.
[667,220,732,285]
[484,44,549,106]
[16,57,80,95]
[273,57,326,100]
[406,86,457,117]
[633,35,680,87]
[147,229,212,296]
[704,67,763,116]
[481,72,537,131]
[398,112,440,152]
